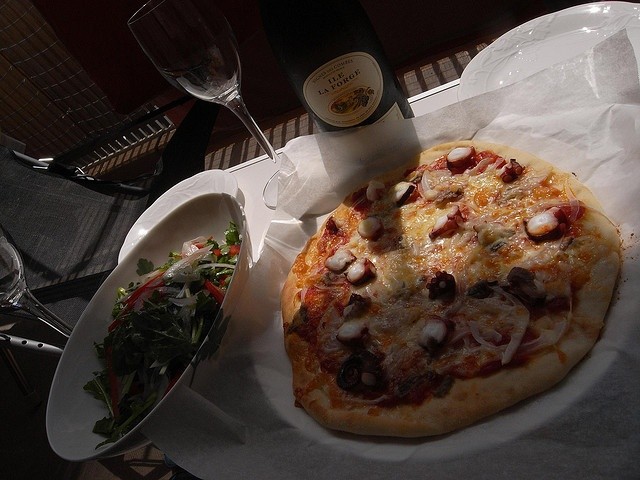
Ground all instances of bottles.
[277,1,419,192]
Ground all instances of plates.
[42,190,254,463]
[117,168,239,264]
[457,0,639,104]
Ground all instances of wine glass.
[0,234,73,337]
[126,0,317,211]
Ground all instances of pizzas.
[279,141,621,438]
